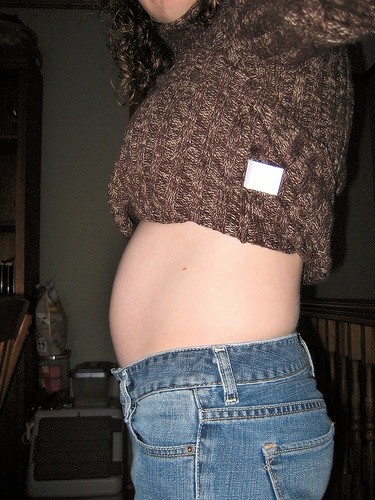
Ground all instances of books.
[70,361,115,379]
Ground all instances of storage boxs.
[23,399,125,497]
[69,361,119,408]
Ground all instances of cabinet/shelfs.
[1,61,44,466]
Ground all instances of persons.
[103,0,375,500]
